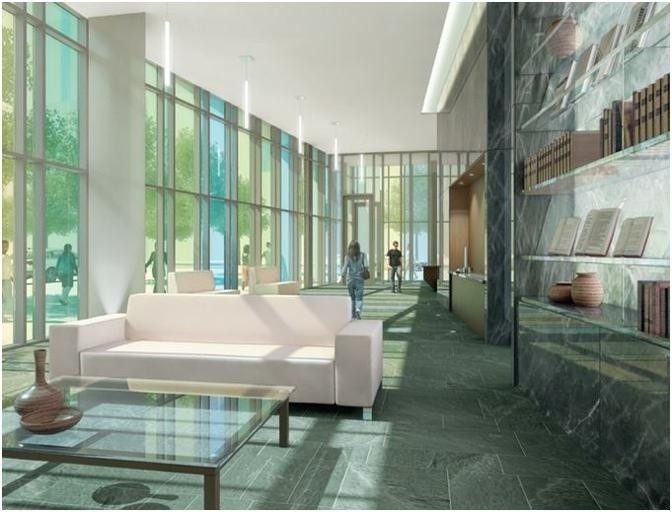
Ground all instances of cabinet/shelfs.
[514,2,670,351]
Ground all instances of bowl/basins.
[20,407,83,434]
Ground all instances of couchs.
[248,267,301,295]
[49,294,384,419]
[167,269,241,294]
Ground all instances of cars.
[11,248,78,284]
